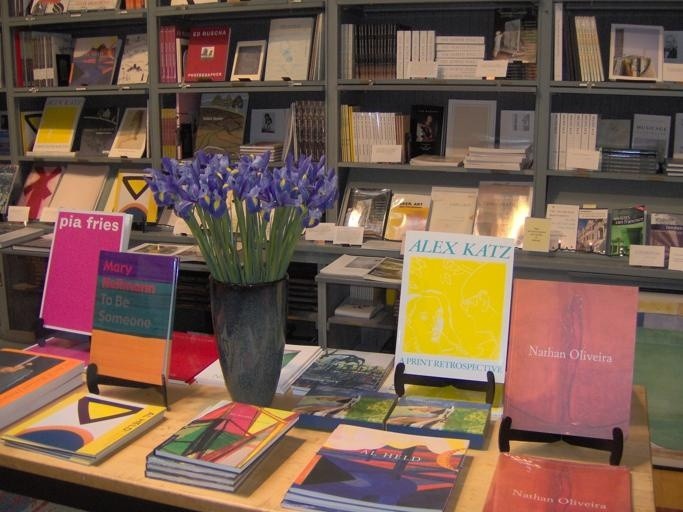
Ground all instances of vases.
[208,272,291,409]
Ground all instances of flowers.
[143,147,339,282]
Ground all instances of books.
[335,286,395,319]
[657,31,683,84]
[554,3,605,85]
[296,347,393,391]
[472,178,534,249]
[8,0,147,15]
[193,343,324,397]
[39,209,134,337]
[0,388,166,467]
[609,206,647,258]
[19,96,150,160]
[482,452,633,512]
[577,208,608,255]
[411,103,442,156]
[13,29,70,86]
[144,398,299,493]
[409,155,460,168]
[608,23,664,82]
[549,111,683,177]
[0,347,86,436]
[340,103,407,165]
[319,252,401,285]
[161,93,325,165]
[635,291,683,472]
[343,187,392,241]
[650,212,683,260]
[280,424,470,512]
[546,203,579,252]
[19,163,158,227]
[502,278,638,442]
[462,143,532,170]
[292,386,492,444]
[159,12,325,82]
[116,32,150,85]
[128,243,252,264]
[428,186,478,235]
[69,37,122,85]
[90,250,179,386]
[499,109,533,144]
[168,329,219,388]
[444,98,496,161]
[340,6,538,81]
[394,232,516,385]
[384,193,431,242]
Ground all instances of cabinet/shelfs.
[316,255,401,349]
[2,1,681,347]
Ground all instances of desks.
[0,329,681,512]
